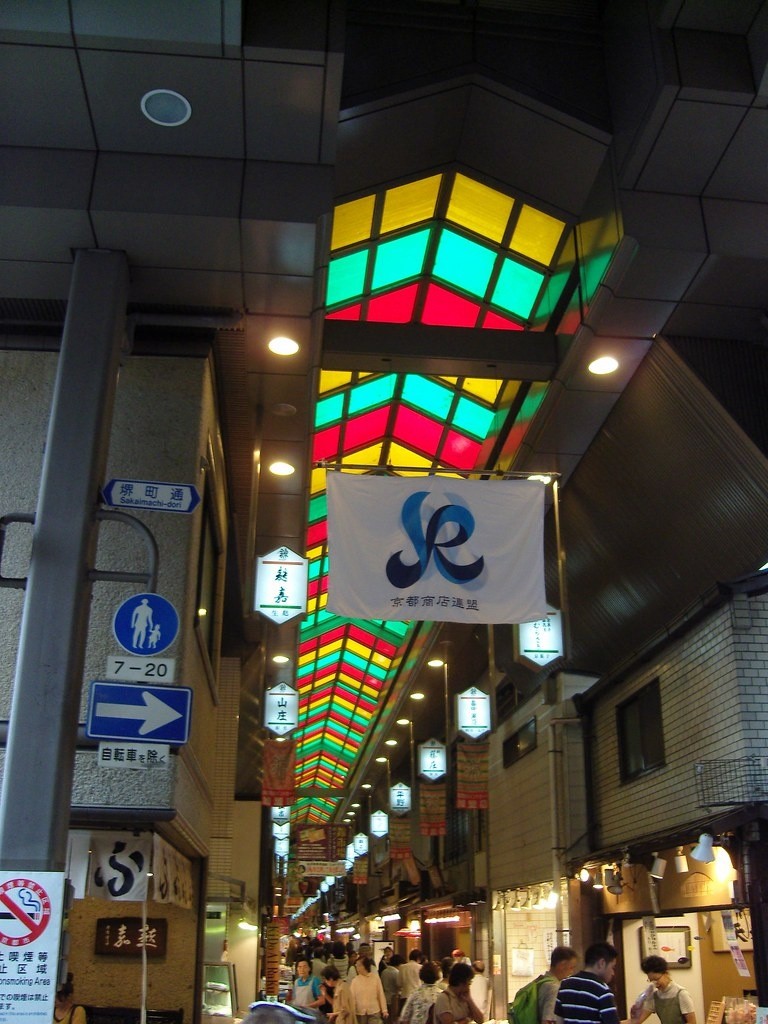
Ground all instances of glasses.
[647,973,666,984]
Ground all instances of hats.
[452,950,465,957]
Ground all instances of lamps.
[238,911,258,930]
[494,883,561,911]
[425,909,460,923]
[569,833,715,894]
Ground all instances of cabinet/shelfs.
[201,962,239,1024]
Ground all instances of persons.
[619,955,697,1024]
[285,934,492,1024]
[553,942,620,1024]
[52,982,86,1024]
[536,946,577,1024]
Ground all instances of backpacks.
[506,974,554,1024]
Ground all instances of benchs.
[73,1004,184,1024]
